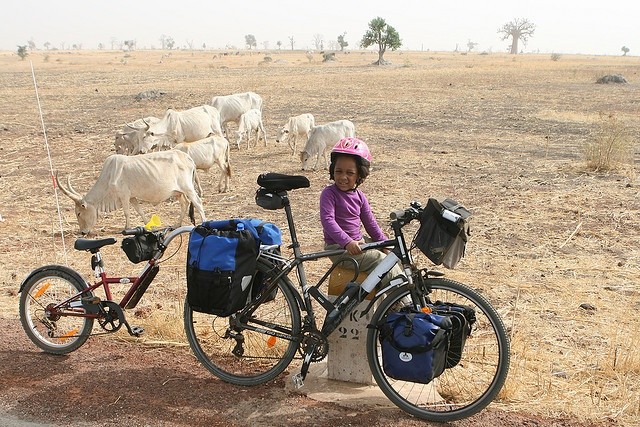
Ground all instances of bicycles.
[19,225,197,355]
[185,173,510,422]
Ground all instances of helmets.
[331,137,372,166]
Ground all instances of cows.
[173,136,233,197]
[54,149,206,236]
[298,120,356,171]
[124,105,225,155]
[275,113,315,157]
[233,108,267,150]
[211,91,263,142]
[113,117,161,156]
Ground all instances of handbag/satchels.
[399,300,476,368]
[376,313,453,384]
[121,233,159,264]
[245,218,282,303]
[415,198,472,269]
[186,219,260,317]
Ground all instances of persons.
[320,137,414,308]
